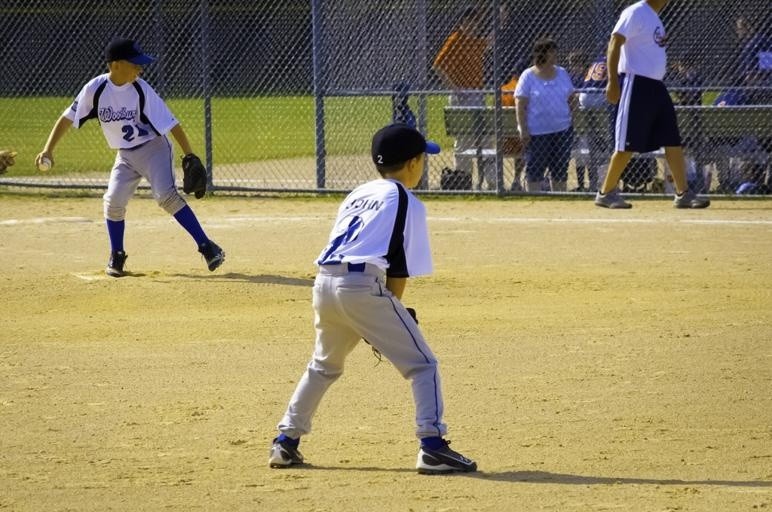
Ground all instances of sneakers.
[416,449,477,476]
[106,252,128,277]
[595,187,632,208]
[673,187,710,208]
[198,240,225,271]
[269,438,304,467]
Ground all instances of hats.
[371,125,441,171]
[105,39,156,65]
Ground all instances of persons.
[661,10,772,194]
[594,0,711,208]
[35,38,225,277]
[268,123,478,474]
[431,4,655,191]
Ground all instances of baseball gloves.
[181,153,206,199]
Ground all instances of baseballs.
[38,157,51,171]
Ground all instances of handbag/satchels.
[440,165,472,189]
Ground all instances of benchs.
[443,105,772,191]
